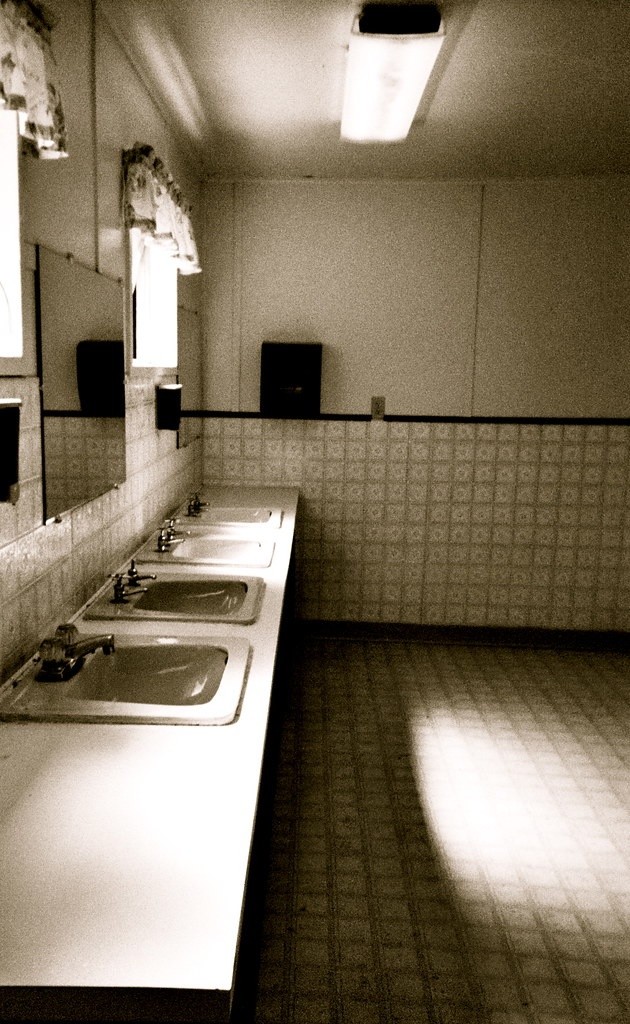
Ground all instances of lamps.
[341,2,446,143]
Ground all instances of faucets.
[133,572,156,580]
[193,492,210,508]
[128,559,157,585]
[109,572,149,599]
[188,499,208,516]
[164,517,192,540]
[156,527,185,550]
[55,625,116,656]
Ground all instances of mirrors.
[34,242,126,525]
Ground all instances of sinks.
[62,636,250,725]
[173,538,274,568]
[134,572,264,626]
[201,507,282,530]
